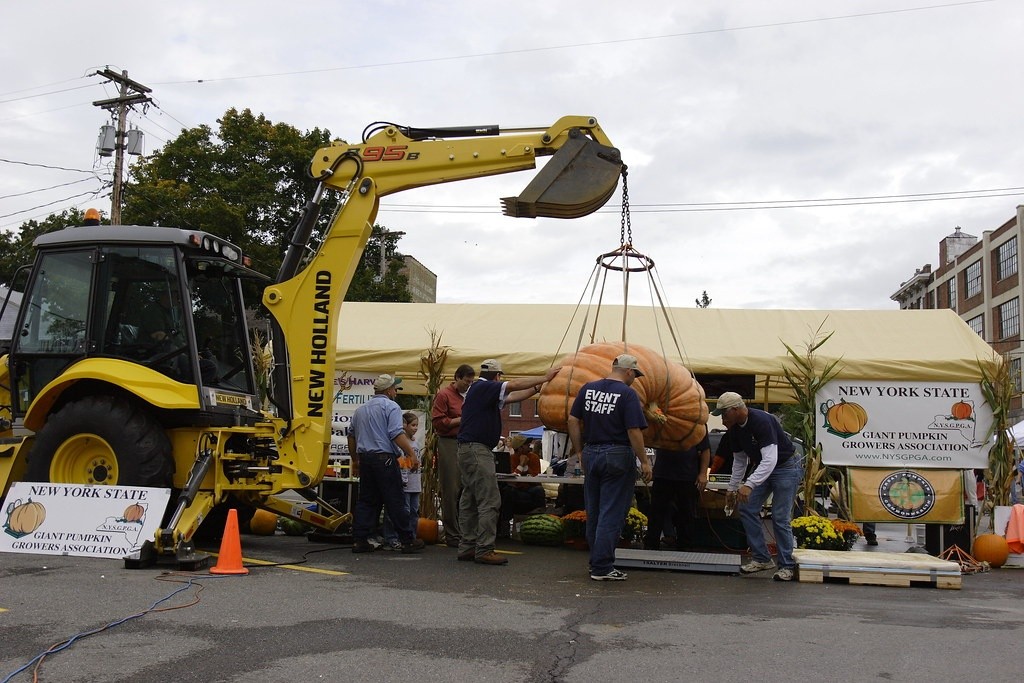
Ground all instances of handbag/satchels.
[964,475,985,501]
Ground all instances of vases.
[564,538,588,550]
[615,539,631,549]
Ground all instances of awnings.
[519,426,546,439]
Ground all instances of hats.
[374,373,396,391]
[712,392,745,416]
[511,436,533,452]
[612,354,644,377]
[480,358,504,375]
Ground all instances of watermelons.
[519,514,566,546]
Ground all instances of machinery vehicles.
[0,115,624,570]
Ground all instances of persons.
[136,277,188,355]
[492,435,515,455]
[431,365,475,548]
[501,436,546,515]
[974,458,1024,515]
[456,359,563,565]
[568,354,652,581]
[711,392,802,581]
[837,466,878,545]
[347,373,424,555]
[642,424,754,552]
[530,441,542,459]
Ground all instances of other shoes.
[458,550,507,564]
[866,533,878,545]
[352,538,374,553]
[401,538,424,554]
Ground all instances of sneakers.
[772,568,796,581]
[741,559,775,573]
[589,567,628,581]
[382,538,401,550]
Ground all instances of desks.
[318,477,359,515]
[496,474,776,552]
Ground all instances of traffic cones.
[209,508,250,575]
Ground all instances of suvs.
[707,428,815,482]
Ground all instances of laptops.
[493,452,512,474]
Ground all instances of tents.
[993,420,1024,450]
[262,301,1007,563]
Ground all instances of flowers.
[790,515,845,550]
[826,519,864,551]
[560,510,587,539]
[619,507,648,543]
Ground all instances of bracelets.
[533,386,539,393]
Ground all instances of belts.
[440,435,457,439]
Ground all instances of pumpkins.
[249,509,278,535]
[418,517,438,544]
[538,342,708,452]
[974,533,1009,568]
